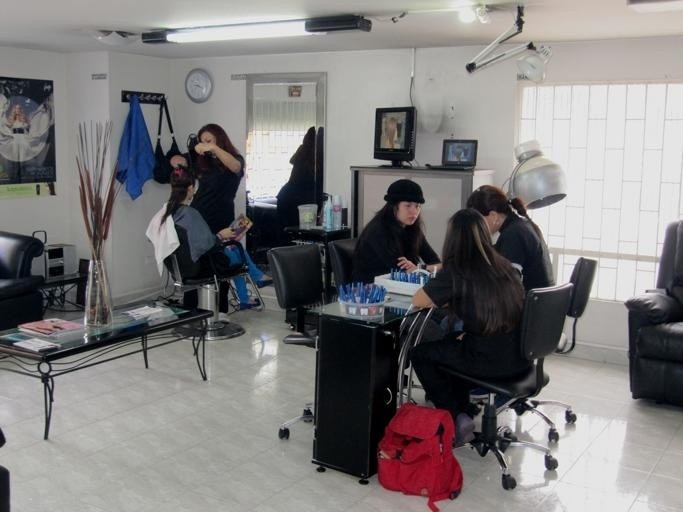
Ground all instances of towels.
[115,93,157,201]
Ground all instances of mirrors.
[241,72,327,287]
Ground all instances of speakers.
[32,244,77,280]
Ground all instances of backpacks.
[151,138,183,185]
[375,402,463,500]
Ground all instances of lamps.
[139,14,373,45]
[464,5,554,84]
[86,28,140,48]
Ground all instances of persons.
[6,101,33,184]
[349,177,442,289]
[407,208,533,450]
[383,116,400,149]
[274,126,315,246]
[466,185,555,298]
[151,123,245,325]
[159,163,273,310]
[453,146,467,161]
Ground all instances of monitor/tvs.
[374,106,417,169]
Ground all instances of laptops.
[428,139,477,170]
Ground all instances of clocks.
[183,65,215,103]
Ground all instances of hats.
[383,178,425,205]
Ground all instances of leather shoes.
[238,297,264,310]
[257,276,272,288]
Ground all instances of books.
[225,212,253,242]
[12,338,62,354]
[16,317,83,338]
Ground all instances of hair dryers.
[186,133,213,169]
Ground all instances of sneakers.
[495,394,518,415]
[469,388,496,399]
[454,413,475,445]
[218,313,230,324]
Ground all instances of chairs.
[621,217,683,409]
[263,243,338,442]
[0,226,48,330]
[325,237,426,415]
[435,280,577,489]
[494,254,600,445]
[147,223,264,342]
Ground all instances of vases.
[80,261,117,328]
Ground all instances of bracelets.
[217,233,224,240]
[421,264,426,271]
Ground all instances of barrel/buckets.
[297,204,319,229]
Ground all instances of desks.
[347,162,496,263]
[302,286,448,486]
